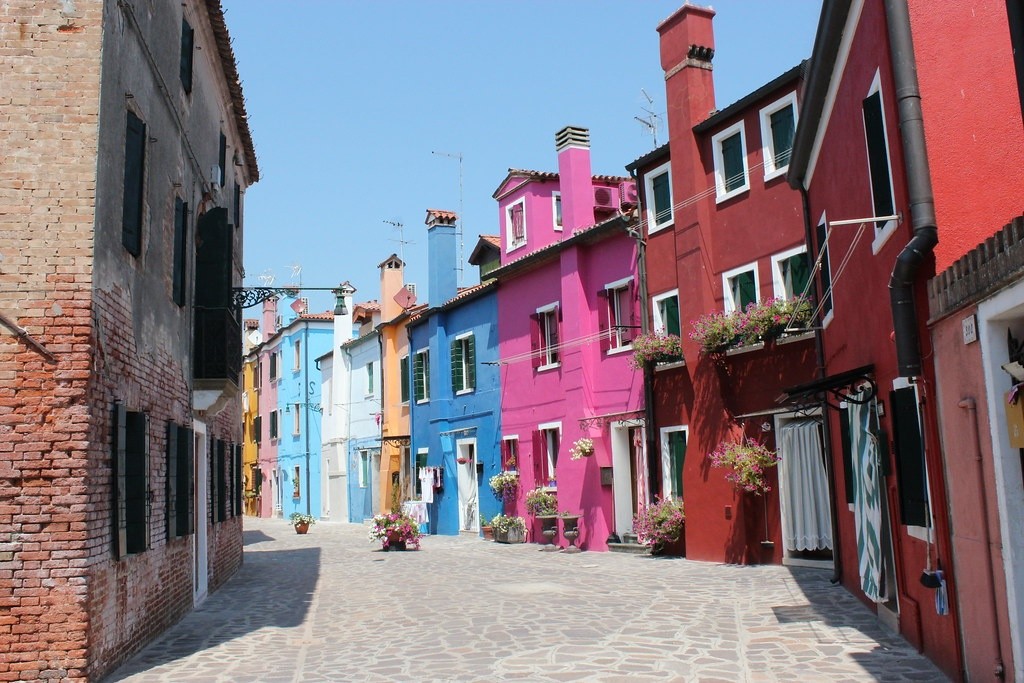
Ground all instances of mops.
[922,403,950,616]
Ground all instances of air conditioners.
[618,181,638,205]
[593,185,619,214]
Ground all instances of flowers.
[686,290,813,354]
[288,512,315,528]
[479,513,488,527]
[366,513,425,551]
[488,470,521,505]
[489,513,529,534]
[568,436,594,461]
[455,458,473,464]
[625,323,684,372]
[706,437,782,498]
[561,510,569,517]
[629,493,686,554]
[546,476,556,481]
[524,488,558,516]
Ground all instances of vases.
[655,521,686,557]
[536,512,563,552]
[561,515,584,553]
[581,449,594,457]
[294,522,309,534]
[458,461,465,465]
[493,526,524,544]
[549,480,556,487]
[294,492,299,497]
[386,527,406,542]
[481,526,494,540]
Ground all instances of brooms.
[918,402,942,588]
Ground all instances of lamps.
[231,282,349,316]
